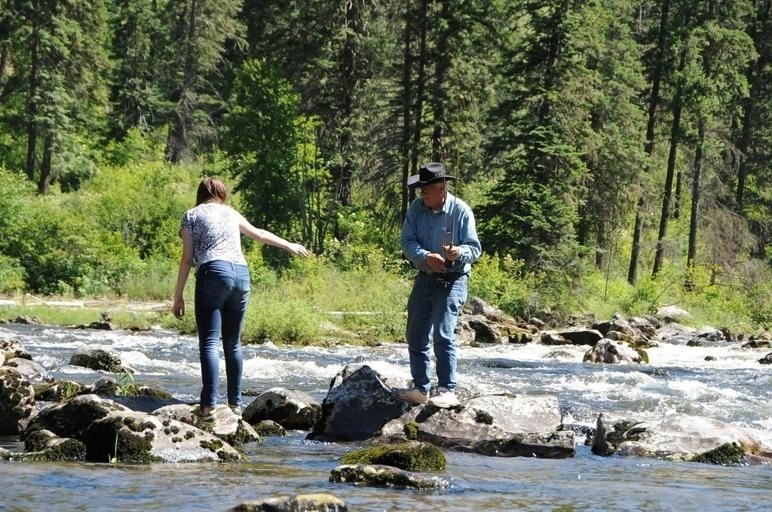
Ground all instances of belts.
[419,270,459,278]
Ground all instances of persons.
[172,176,310,423]
[400,162,483,409]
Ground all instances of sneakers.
[428,391,460,408]
[228,404,241,415]
[190,407,217,427]
[392,386,429,404]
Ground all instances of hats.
[406,162,456,189]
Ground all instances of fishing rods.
[438,38,460,289]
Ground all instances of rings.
[451,256,454,260]
